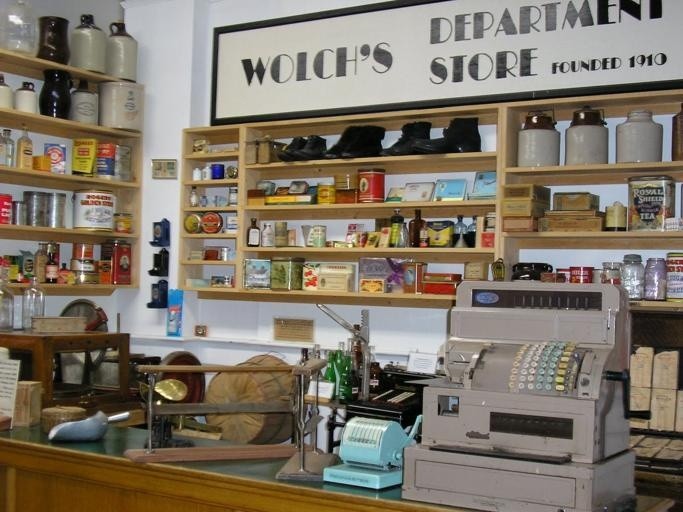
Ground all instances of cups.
[605,206,626,231]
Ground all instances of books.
[385,187,403,202]
[470,170,496,200]
[401,182,435,202]
[432,178,467,201]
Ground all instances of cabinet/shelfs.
[0,46,143,297]
[176,87,682,314]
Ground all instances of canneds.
[11,190,66,228]
[313,225,327,248]
[556,268,571,283]
[592,269,620,283]
[220,248,230,262]
[71,240,132,285]
[229,187,238,207]
[572,267,593,283]
[539,272,555,283]
[665,253,683,302]
[38,241,59,265]
[113,212,133,233]
[317,168,385,205]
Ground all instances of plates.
[185,211,224,234]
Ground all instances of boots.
[379,122,431,156]
[411,117,481,154]
[277,136,307,161]
[319,126,358,159]
[287,135,327,160]
[340,126,385,158]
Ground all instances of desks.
[0,329,129,411]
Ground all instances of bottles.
[255,180,276,195]
[602,251,683,303]
[0,192,66,229]
[247,217,326,248]
[221,247,234,285]
[41,406,87,434]
[0,0,141,171]
[111,211,133,235]
[517,102,683,167]
[369,360,388,394]
[189,162,237,207]
[315,182,335,205]
[300,339,363,404]
[0,242,68,333]
[390,209,478,247]
[346,224,357,244]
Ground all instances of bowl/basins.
[204,352,307,446]
